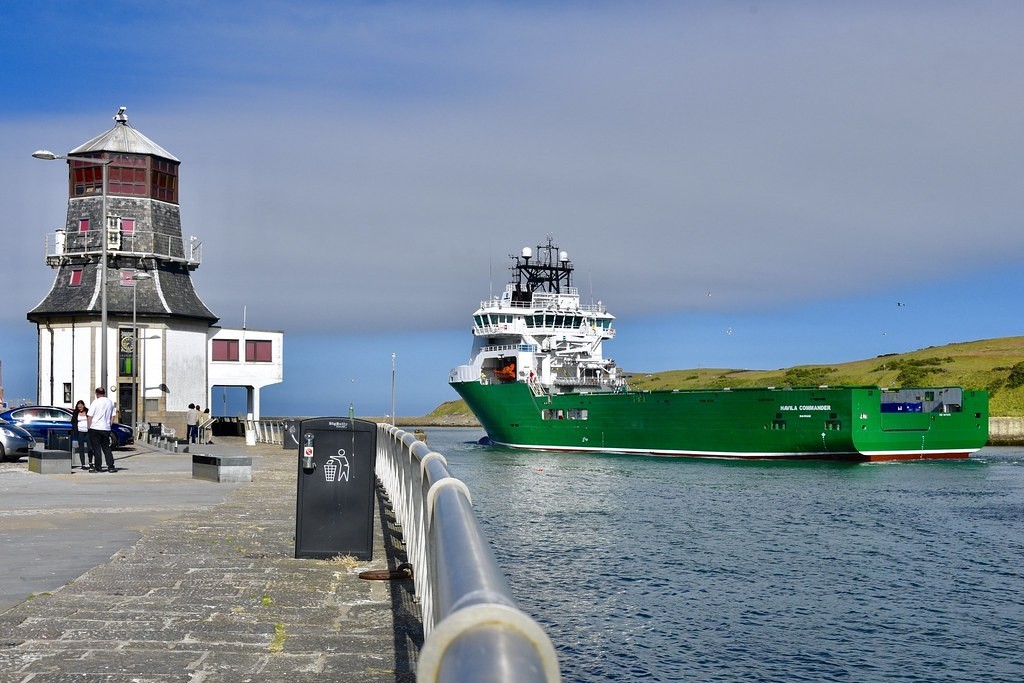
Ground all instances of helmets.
[481,373,484,374]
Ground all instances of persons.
[196,405,203,444]
[186,403,198,444]
[70,401,94,469]
[86,387,117,473]
[202,408,214,444]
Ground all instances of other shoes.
[81,463,95,470]
[108,468,117,473]
[191,441,197,444]
[206,440,213,445]
[89,468,102,472]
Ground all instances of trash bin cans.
[293,415,379,562]
[282,419,300,450]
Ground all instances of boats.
[447,233,989,463]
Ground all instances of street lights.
[32,150,152,400]
[119,282,161,439]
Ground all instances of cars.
[0,406,134,450]
[0,417,36,462]
